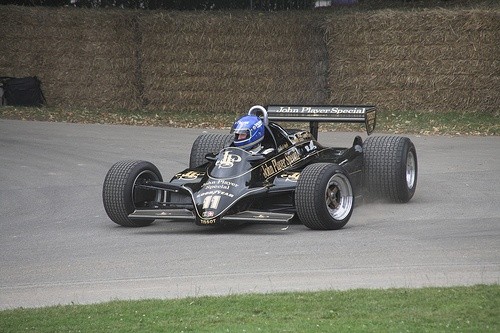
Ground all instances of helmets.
[230,115,265,151]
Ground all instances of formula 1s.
[102,103,418,231]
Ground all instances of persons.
[233,116,275,155]
